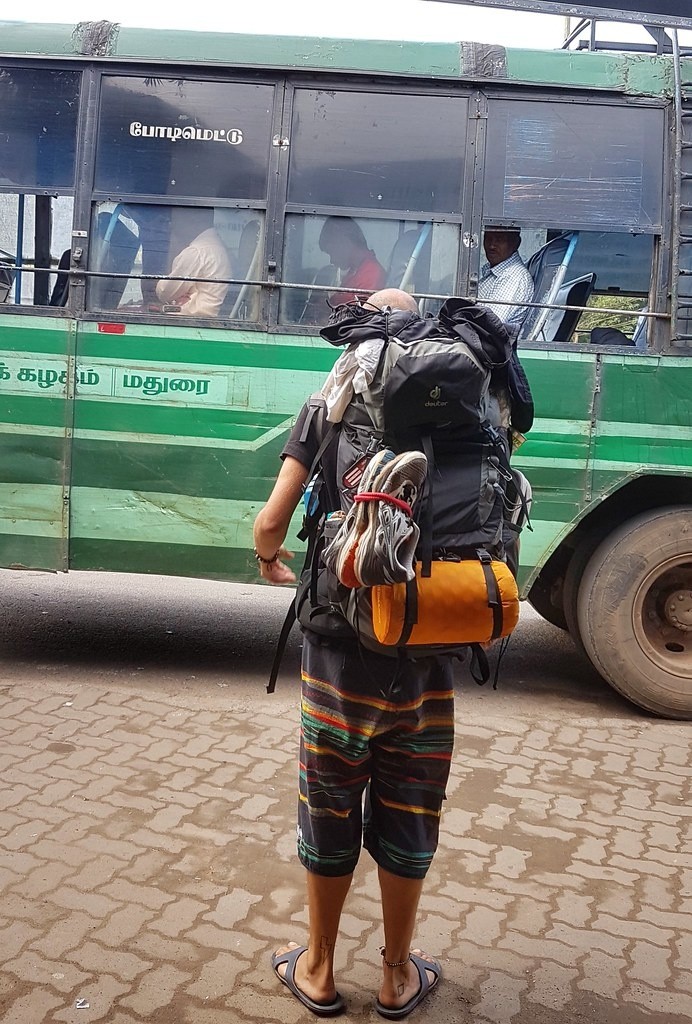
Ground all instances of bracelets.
[254,547,282,573]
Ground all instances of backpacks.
[294,337,533,699]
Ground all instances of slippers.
[374,949,440,1019]
[271,941,345,1016]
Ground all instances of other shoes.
[504,468,531,580]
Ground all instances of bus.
[0,14,692,723]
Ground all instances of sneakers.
[322,447,396,587]
[352,450,428,586]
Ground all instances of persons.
[476,223,535,339]
[253,288,456,1021]
[314,214,388,328]
[156,193,235,319]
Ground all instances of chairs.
[0,201,582,352]
